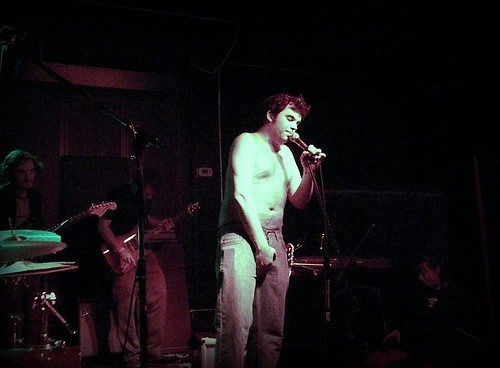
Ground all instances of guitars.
[43,199,117,233]
[101,202,200,275]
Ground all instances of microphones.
[289,132,317,162]
[1,26,32,58]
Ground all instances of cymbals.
[0,260,81,278]
[0,229,62,241]
[0,240,68,259]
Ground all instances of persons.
[97,166,175,367]
[1,150,81,347]
[365,242,493,367]
[214,95,327,368]
